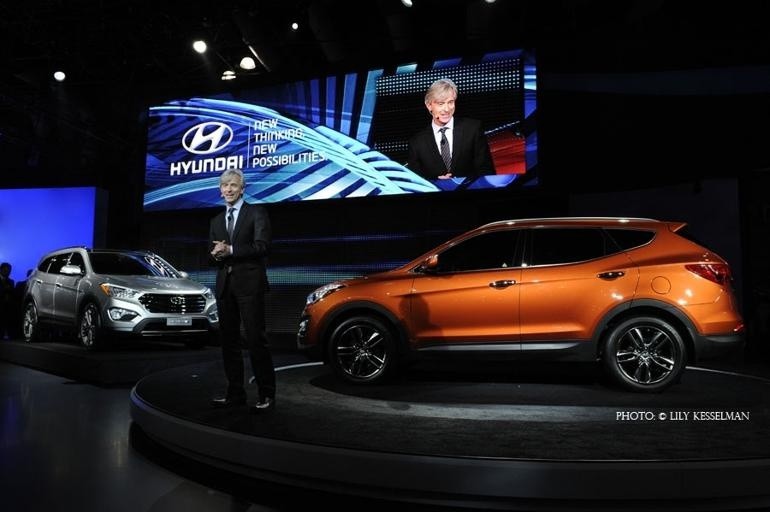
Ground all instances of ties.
[228,208,236,245]
[439,127,452,174]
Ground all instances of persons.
[208,168,277,410]
[0,262,15,340]
[407,78,497,182]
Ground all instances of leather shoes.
[255,397,276,410]
[211,396,247,408]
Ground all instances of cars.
[19,246,219,349]
[296,216,747,393]
